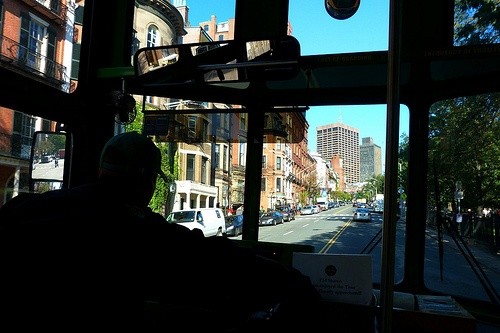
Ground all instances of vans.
[44,155,53,162]
[166,207,226,238]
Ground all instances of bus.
[58,149,65,159]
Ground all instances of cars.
[259,200,383,226]
[32,160,38,170]
[224,214,243,236]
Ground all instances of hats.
[100,131,167,182]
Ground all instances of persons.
[477,210,485,218]
[483,208,500,217]
[292,203,295,209]
[237,204,244,211]
[0,131,323,333]
[54,157,59,168]
[228,206,236,214]
[260,205,265,212]
